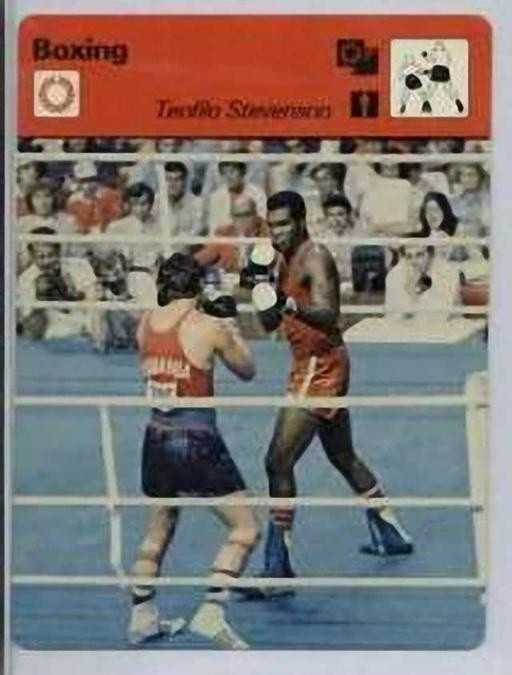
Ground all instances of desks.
[341,317,487,346]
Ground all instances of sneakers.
[361,538,413,555]
[242,578,297,600]
[127,618,186,645]
[188,603,250,650]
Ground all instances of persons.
[123,252,262,650]
[234,190,416,601]
[17,138,491,349]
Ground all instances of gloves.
[247,242,277,275]
[251,283,288,311]
[202,292,237,318]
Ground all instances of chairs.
[12,136,491,304]
